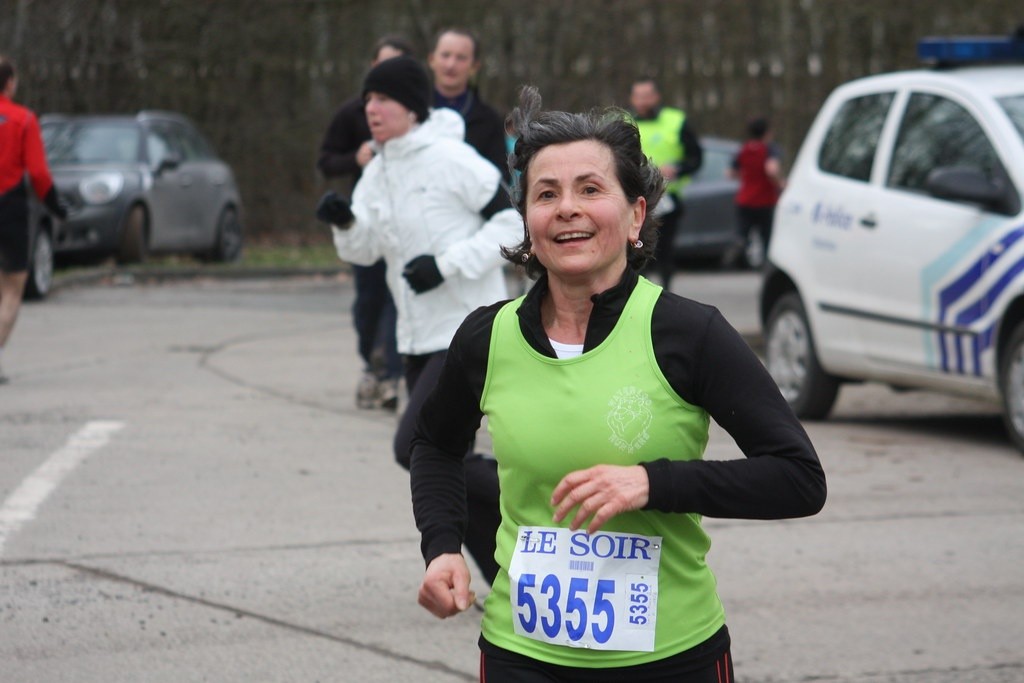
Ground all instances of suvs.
[754,33,1024,448]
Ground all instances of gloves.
[402,254,444,294]
[316,190,353,225]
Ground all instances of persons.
[409,86,827,683]
[313,55,502,589]
[724,118,783,271]
[622,75,703,291]
[0,58,68,382]
[317,38,411,412]
[422,26,522,185]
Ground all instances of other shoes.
[733,254,753,271]
[356,376,376,408]
[373,377,399,408]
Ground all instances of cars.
[666,137,769,270]
[28,106,246,297]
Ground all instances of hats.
[361,55,436,122]
